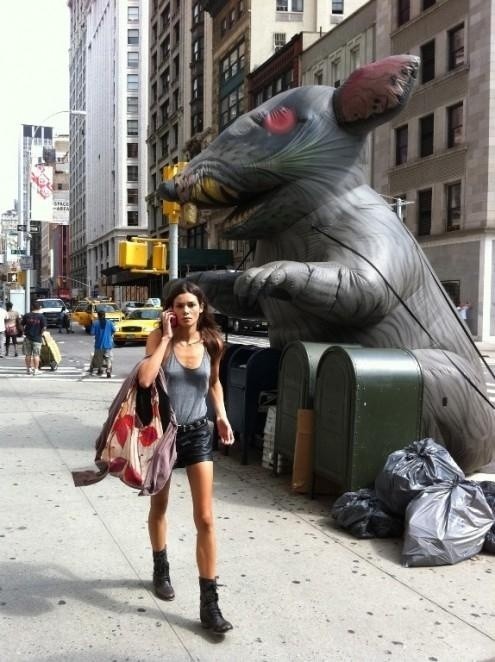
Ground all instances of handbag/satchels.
[100,378,164,489]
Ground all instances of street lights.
[26,110,86,313]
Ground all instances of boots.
[199,577,233,634]
[153,544,175,600]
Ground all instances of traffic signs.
[12,249,25,254]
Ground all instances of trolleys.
[22,334,62,371]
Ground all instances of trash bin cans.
[205,340,425,507]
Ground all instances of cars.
[32,298,69,328]
[64,296,164,346]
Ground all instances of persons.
[4,301,23,357]
[57,306,70,334]
[134,276,237,633]
[89,309,116,378]
[0,300,9,358]
[18,301,49,376]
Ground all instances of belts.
[177,417,208,433]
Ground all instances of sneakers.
[96,369,111,378]
[27,368,43,375]
